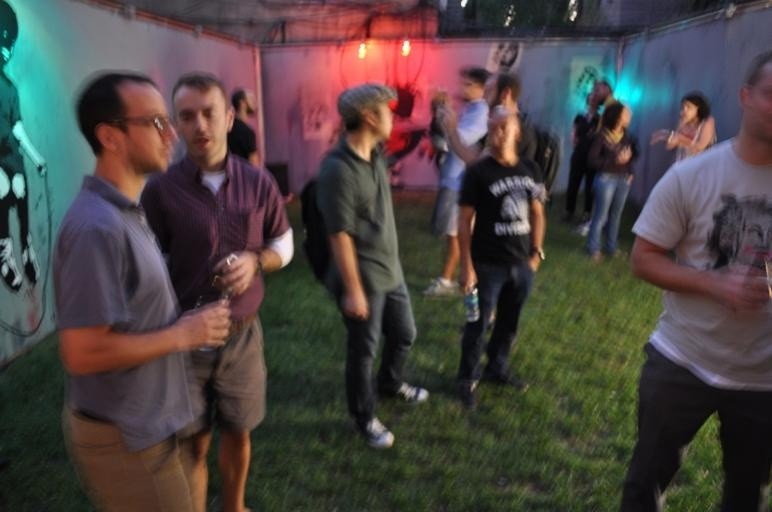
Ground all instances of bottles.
[464,286,480,323]
[745,246,769,277]
[194,274,227,352]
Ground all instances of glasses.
[111,116,163,131]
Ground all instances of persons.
[315,82,429,448]
[50,68,233,512]
[619,51,772,512]
[226,89,262,167]
[570,84,641,259]
[428,69,534,291]
[138,72,294,512]
[454,106,551,411]
[651,92,718,164]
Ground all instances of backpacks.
[301,152,359,279]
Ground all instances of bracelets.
[251,250,264,270]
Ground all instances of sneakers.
[396,382,430,403]
[424,277,452,297]
[361,417,395,449]
[462,375,529,407]
